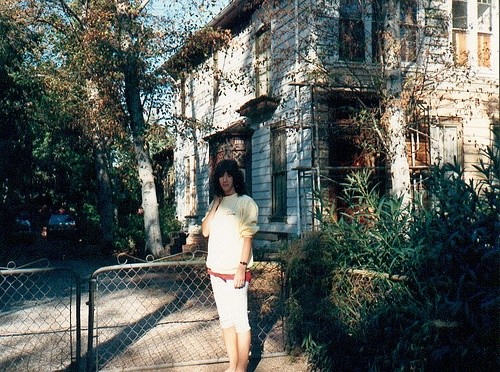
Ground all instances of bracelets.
[240,261,247,265]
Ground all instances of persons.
[200,158,260,372]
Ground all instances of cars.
[46,214,77,240]
